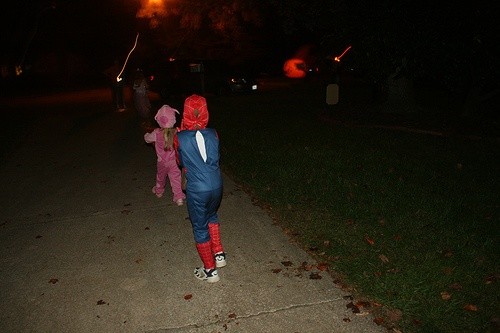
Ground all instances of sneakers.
[214,251,227,268]
[194,266,220,282]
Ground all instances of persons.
[174,93,228,284]
[102,58,153,125]
[142,104,186,207]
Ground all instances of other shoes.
[152,186,162,197]
[176,198,184,205]
